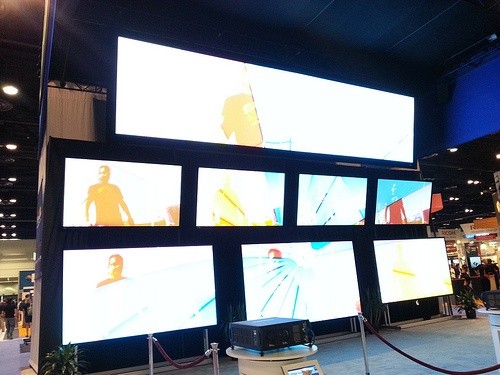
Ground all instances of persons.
[384,183,407,224]
[85,165,134,225]
[476,259,500,280]
[451,264,471,286]
[24,298,32,338]
[96,255,123,288]
[0,297,16,339]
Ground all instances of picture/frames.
[281,357,324,375]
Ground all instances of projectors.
[229,317,313,351]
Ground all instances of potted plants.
[453,279,479,319]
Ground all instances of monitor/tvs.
[470,257,482,268]
[60,156,455,347]
